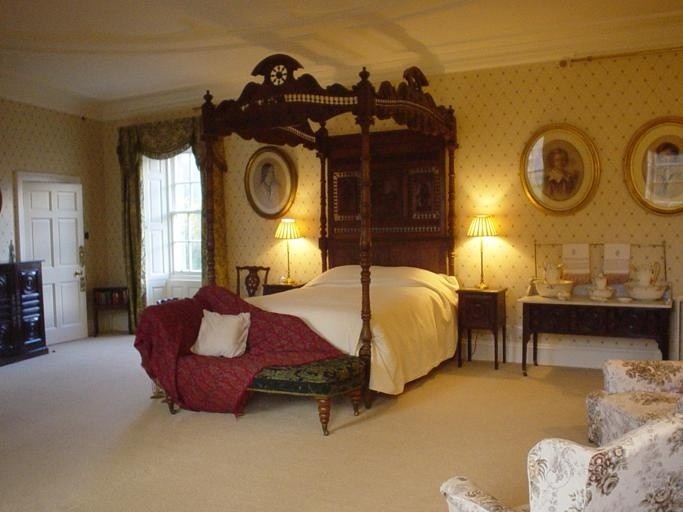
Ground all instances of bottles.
[8,240,15,264]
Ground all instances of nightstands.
[262,278,310,295]
[454,286,509,371]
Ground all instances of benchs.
[134,282,366,434]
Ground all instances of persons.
[653,143,683,198]
[254,164,284,209]
[541,149,575,201]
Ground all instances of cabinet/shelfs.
[0,260,48,366]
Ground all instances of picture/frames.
[622,115,683,218]
[518,123,602,216]
[244,145,297,219]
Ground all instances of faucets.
[556,262,565,280]
[648,261,660,284]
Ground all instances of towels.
[602,242,630,274]
[561,241,590,276]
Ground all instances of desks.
[91,286,134,336]
[516,294,675,377]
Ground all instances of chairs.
[439,412,682,512]
[236,265,270,297]
[583,358,682,448]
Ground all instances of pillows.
[189,307,252,360]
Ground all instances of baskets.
[534,266,574,299]
[625,263,668,300]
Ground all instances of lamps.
[275,219,303,284]
[466,215,498,290]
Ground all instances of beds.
[200,50,459,414]
